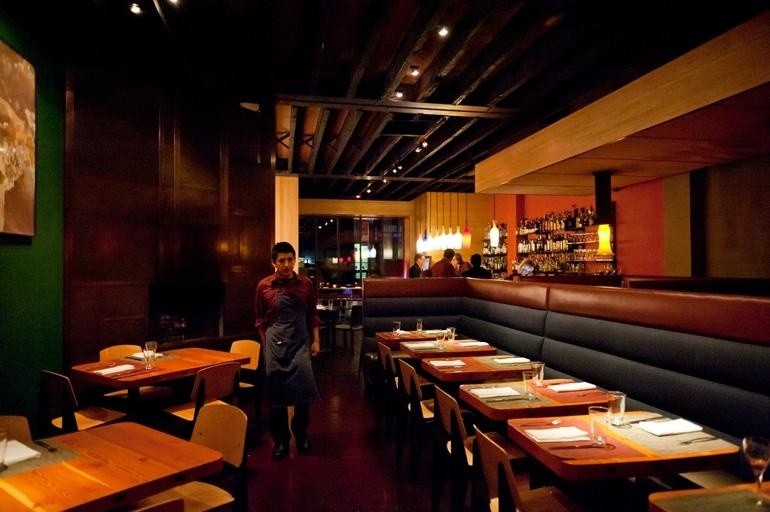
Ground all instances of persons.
[459,253,492,279]
[429,248,457,278]
[254,242,323,458]
[453,252,472,276]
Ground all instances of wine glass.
[741,431,770,509]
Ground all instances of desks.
[399,339,498,358]
[507,410,741,497]
[649,480,770,511]
[375,329,458,350]
[422,354,542,383]
[459,378,621,420]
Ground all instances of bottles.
[481,203,614,275]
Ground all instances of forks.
[515,419,561,428]
[675,436,720,446]
[33,440,58,453]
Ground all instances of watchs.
[408,254,426,278]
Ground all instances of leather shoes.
[295,435,312,454]
[271,440,289,459]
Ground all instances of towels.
[0,438,42,466]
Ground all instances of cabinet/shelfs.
[481,208,614,277]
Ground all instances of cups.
[0,424,9,468]
[522,372,538,402]
[417,319,424,333]
[606,391,627,425]
[316,297,333,310]
[435,331,447,351]
[392,320,401,337]
[145,341,158,352]
[140,346,155,371]
[531,361,545,386]
[588,404,610,446]
[445,327,457,343]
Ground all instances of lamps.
[413,114,502,257]
[590,173,614,259]
[391,20,454,101]
[316,136,431,232]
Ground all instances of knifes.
[485,397,539,404]
[544,445,600,450]
[627,416,664,425]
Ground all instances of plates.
[133,352,163,360]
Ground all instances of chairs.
[434,384,528,473]
[39,369,128,435]
[226,338,267,429]
[127,403,249,510]
[376,341,434,394]
[158,361,241,442]
[0,415,32,441]
[130,495,185,512]
[311,275,362,354]
[471,423,581,512]
[398,358,471,428]
[98,342,173,423]
